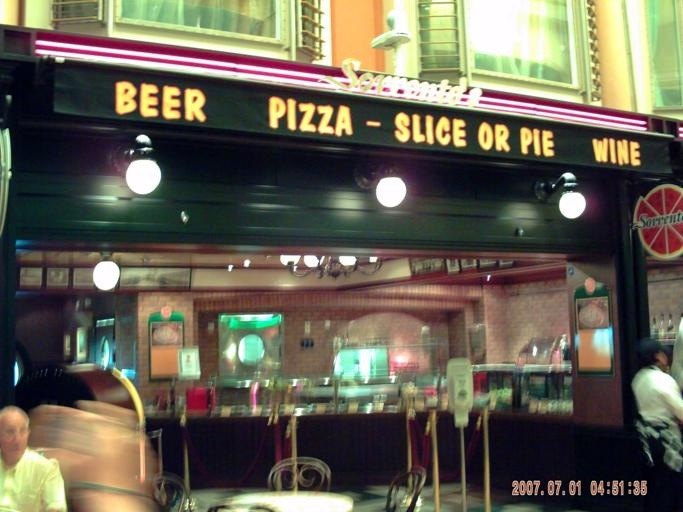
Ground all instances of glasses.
[0,426,32,436]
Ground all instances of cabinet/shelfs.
[170,362,574,485]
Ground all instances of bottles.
[652,313,675,342]
[560,334,569,360]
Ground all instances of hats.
[639,336,673,355]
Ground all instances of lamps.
[122,135,163,196]
[225,250,384,284]
[93,251,122,289]
[351,155,408,211]
[532,169,588,220]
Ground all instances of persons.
[628,334,682,511]
[0,406,70,511]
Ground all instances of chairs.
[149,456,430,512]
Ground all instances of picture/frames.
[119,267,191,293]
[12,262,96,291]
[407,255,518,279]
